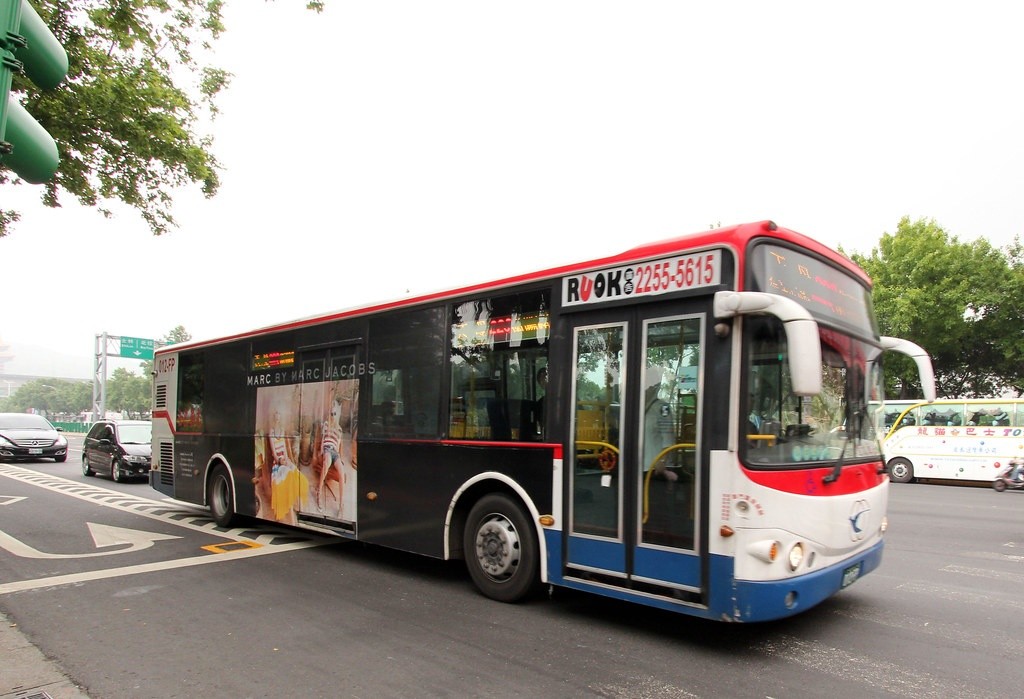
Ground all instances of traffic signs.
[120,335,154,361]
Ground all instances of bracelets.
[278,457,282,466]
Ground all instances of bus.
[145,218,938,639]
[828,397,1024,495]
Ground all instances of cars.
[0,412,68,462]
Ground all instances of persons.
[535,367,547,442]
[269,405,309,526]
[317,400,344,519]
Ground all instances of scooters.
[993,457,1024,492]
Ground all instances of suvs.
[80,418,153,483]
